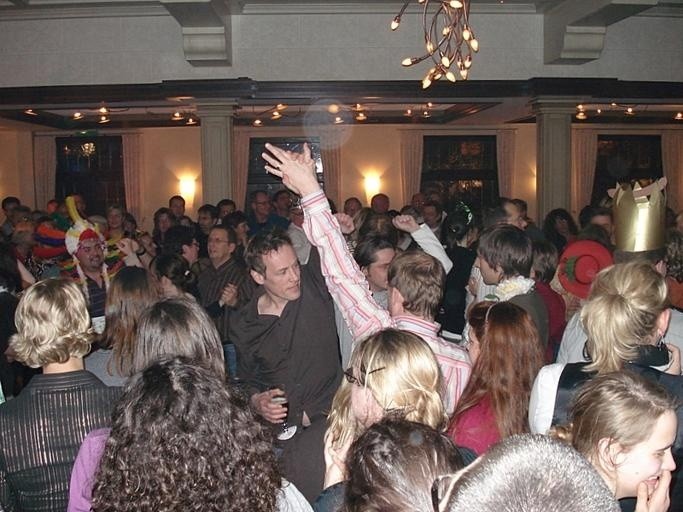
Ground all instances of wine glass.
[267,384,297,441]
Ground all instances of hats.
[64,219,108,257]
[559,241,615,299]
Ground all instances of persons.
[0,143,682,511]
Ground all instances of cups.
[90,316,107,334]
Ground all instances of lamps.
[403,103,437,119]
[391,0,480,91]
[74,106,130,124]
[171,108,198,125]
[327,103,368,124]
[251,104,288,128]
[575,103,638,120]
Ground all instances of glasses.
[344,364,363,388]
[256,201,272,205]
[207,237,230,243]
